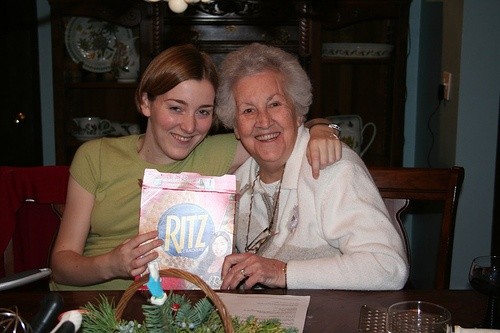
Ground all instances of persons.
[49,44,343,290]
[214,42,410,292]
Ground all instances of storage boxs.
[139,168,241,290]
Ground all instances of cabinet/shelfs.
[47,0,411,165]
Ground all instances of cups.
[385,300,453,333]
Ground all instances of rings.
[240,267,248,278]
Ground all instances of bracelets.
[282,263,287,289]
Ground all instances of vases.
[112,36,140,82]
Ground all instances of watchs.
[309,123,342,137]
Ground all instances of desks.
[0,289,500,333]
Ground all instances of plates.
[64,16,133,73]
[75,134,106,141]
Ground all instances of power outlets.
[442,72,452,100]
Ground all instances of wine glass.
[468,256,500,329]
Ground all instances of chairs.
[0,167,70,280]
[368,167,464,289]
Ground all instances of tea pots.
[328,116,376,158]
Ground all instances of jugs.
[111,35,141,83]
[73,117,111,135]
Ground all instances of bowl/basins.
[320,43,396,57]
